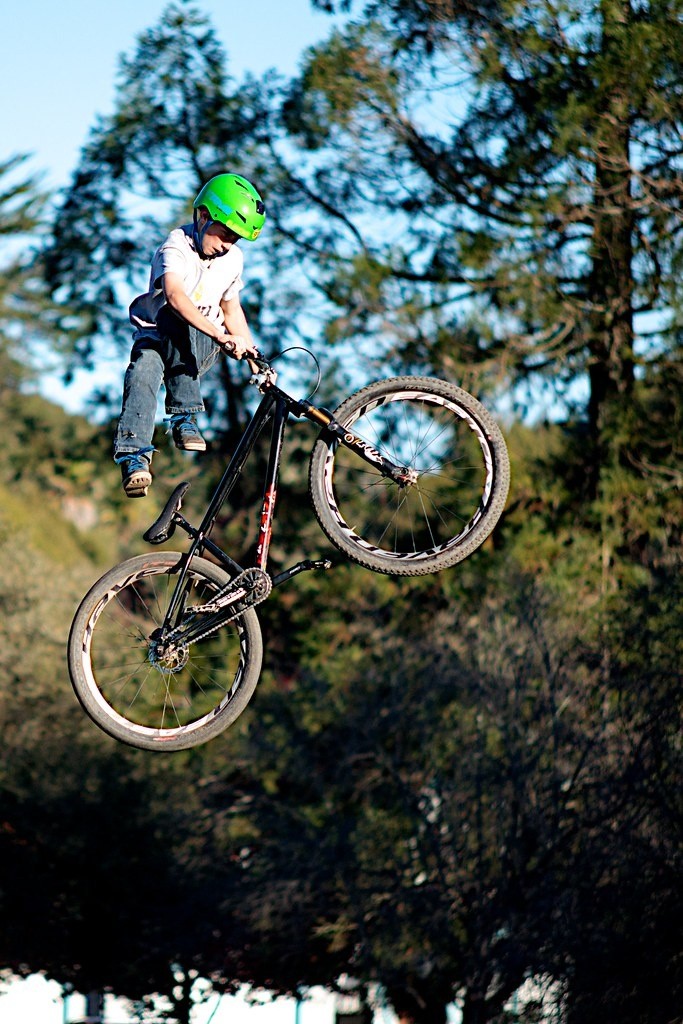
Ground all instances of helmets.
[193,173,267,242]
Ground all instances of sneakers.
[162,412,206,451]
[116,444,155,497]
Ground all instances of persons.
[111,172,280,499]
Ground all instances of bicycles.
[67,340,512,753]
